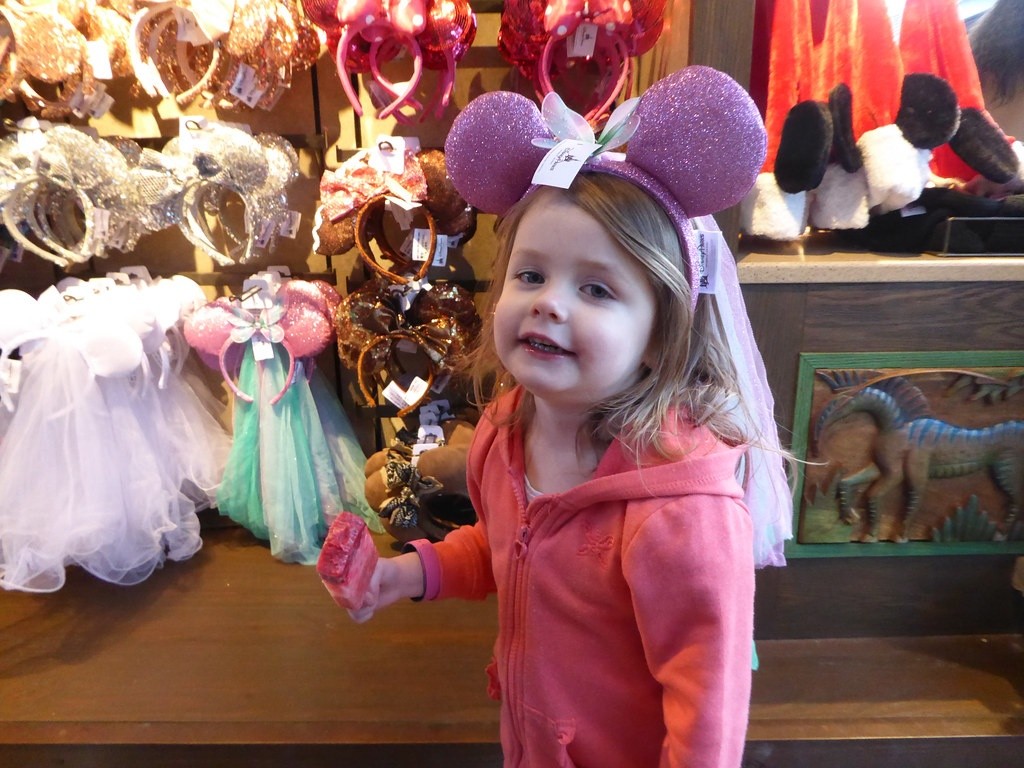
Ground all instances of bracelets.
[402,539,439,604]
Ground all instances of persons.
[343,160,755,768]
